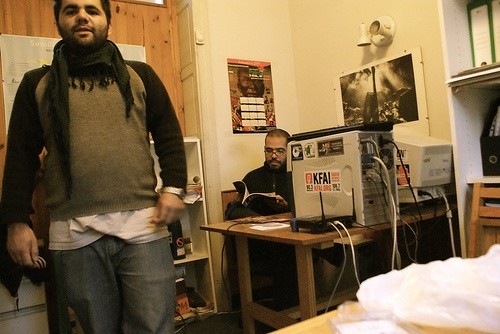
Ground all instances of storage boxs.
[173,276,188,318]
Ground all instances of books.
[232,180,277,207]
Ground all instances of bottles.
[168,219,186,260]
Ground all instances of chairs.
[221,188,242,325]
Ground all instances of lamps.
[357,15,395,49]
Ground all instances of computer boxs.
[287,131,401,228]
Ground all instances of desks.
[200,202,458,334]
[265,300,499,334]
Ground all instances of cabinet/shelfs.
[154,135,218,324]
[436,0,500,257]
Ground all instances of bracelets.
[161,186,186,201]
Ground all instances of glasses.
[264,150,287,157]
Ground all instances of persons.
[229,65,275,132]
[225,129,297,329]
[0,0,187,334]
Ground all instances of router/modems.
[290,188,356,232]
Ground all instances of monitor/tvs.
[391,131,453,204]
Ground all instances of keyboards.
[287,121,395,141]
